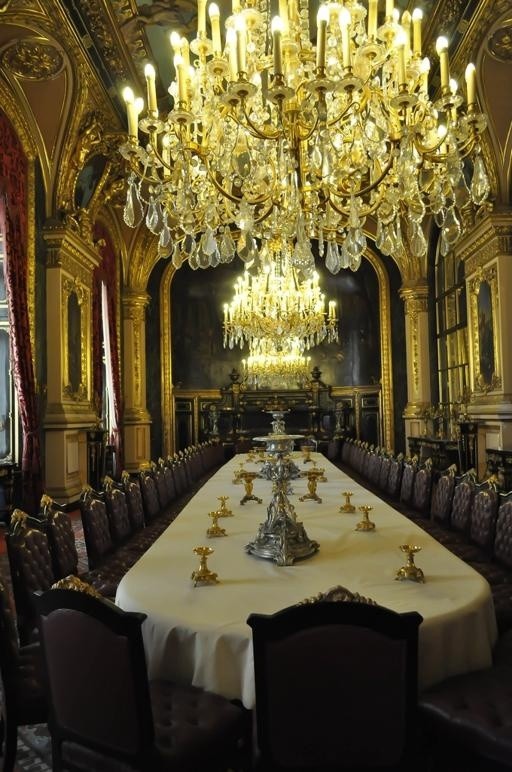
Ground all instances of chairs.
[246,585,424,770]
[30,575,252,771]
[2,585,50,770]
[0,432,509,646]
[418,667,510,772]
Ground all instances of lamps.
[114,0,491,396]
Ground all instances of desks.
[114,452,491,709]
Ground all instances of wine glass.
[191,443,426,584]
